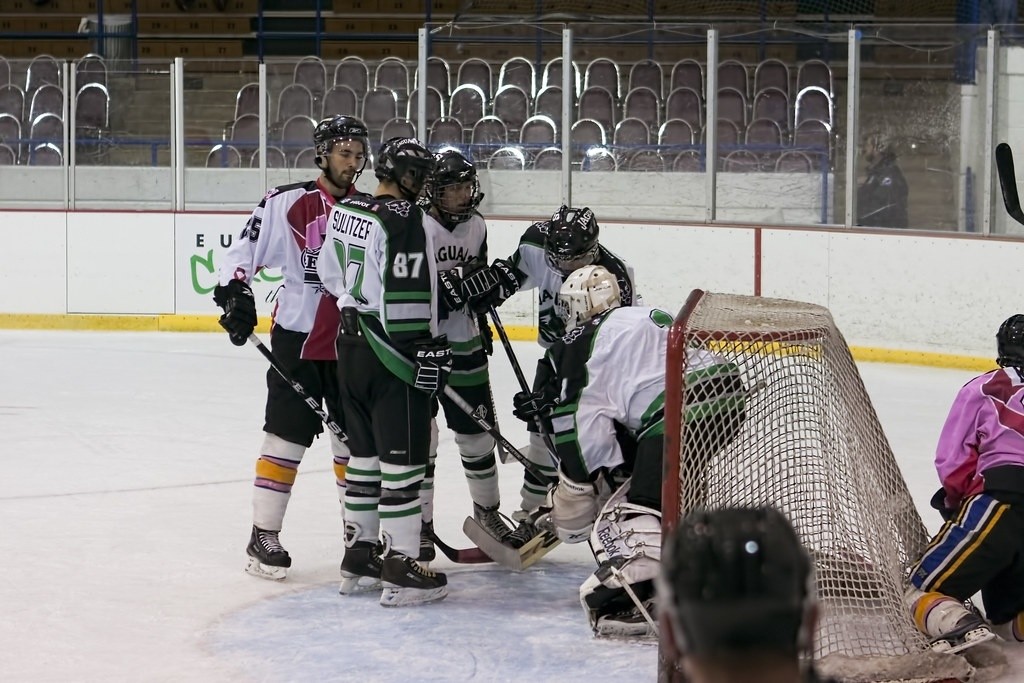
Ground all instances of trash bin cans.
[87,14,134,77]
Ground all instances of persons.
[462,203,638,548]
[656,504,817,682]
[852,119,908,228]
[313,138,454,591]
[412,145,512,560]
[904,315,1023,657]
[513,264,745,639]
[212,114,374,573]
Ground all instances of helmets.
[655,507,815,659]
[543,205,600,261]
[994,315,1024,368]
[312,114,371,149]
[553,265,620,334]
[424,146,485,223]
[374,137,437,211]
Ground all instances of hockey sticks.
[249,332,493,563]
[487,303,561,468]
[443,383,553,487]
[462,378,768,571]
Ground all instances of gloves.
[512,384,559,423]
[438,254,488,312]
[213,279,257,346]
[414,332,453,401]
[540,307,567,349]
[930,486,960,523]
[460,258,528,315]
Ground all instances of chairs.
[76,83,110,164]
[223,82,271,142]
[29,84,63,130]
[230,112,259,154]
[27,142,64,166]
[320,84,359,123]
[274,83,314,131]
[250,145,287,169]
[334,55,369,102]
[26,54,60,95]
[0,57,25,165]
[294,147,326,168]
[205,143,242,168]
[74,53,108,97]
[361,53,836,175]
[293,55,327,104]
[282,115,318,155]
[29,113,63,153]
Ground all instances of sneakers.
[380,529,449,608]
[921,607,1010,680]
[1013,614,1024,642]
[507,506,548,549]
[245,524,292,582]
[415,519,436,569]
[338,520,386,595]
[473,501,517,544]
